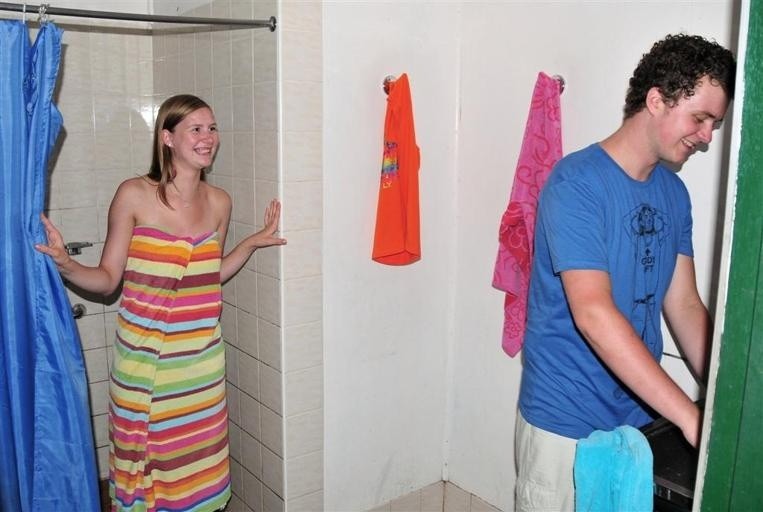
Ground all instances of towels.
[106,226,236,511]
[573,427,654,512]
[491,74,564,355]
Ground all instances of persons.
[32,94,290,511]
[510,32,738,512]
